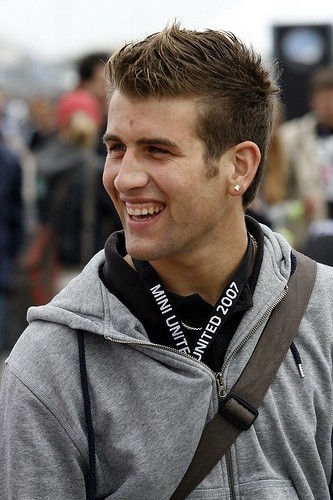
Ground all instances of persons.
[0,20,333,500]
[279,69,333,264]
[61,52,112,137]
